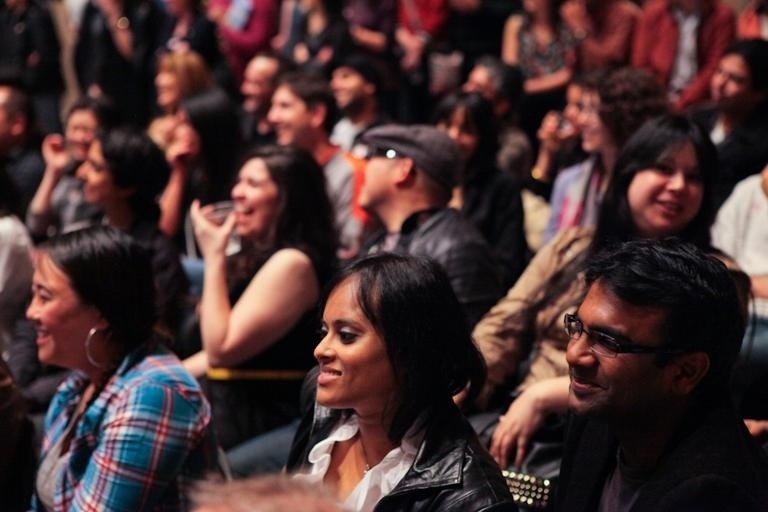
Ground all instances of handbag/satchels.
[476,414,572,511]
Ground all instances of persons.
[180,145,334,453]
[540,68,660,247]
[556,244,768,512]
[682,35,768,197]
[27,104,109,244]
[24,228,217,511]
[449,119,730,499]
[1,219,45,398]
[266,81,384,265]
[286,251,513,512]
[151,92,259,254]
[209,120,512,482]
[708,163,767,415]
[531,75,610,200]
[23,132,190,422]
[425,101,528,295]
[1,1,768,135]
[0,87,40,224]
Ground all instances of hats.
[362,125,464,192]
[324,54,384,92]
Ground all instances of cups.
[551,115,576,142]
[201,203,241,257]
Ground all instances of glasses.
[365,149,420,180]
[564,312,692,360]
